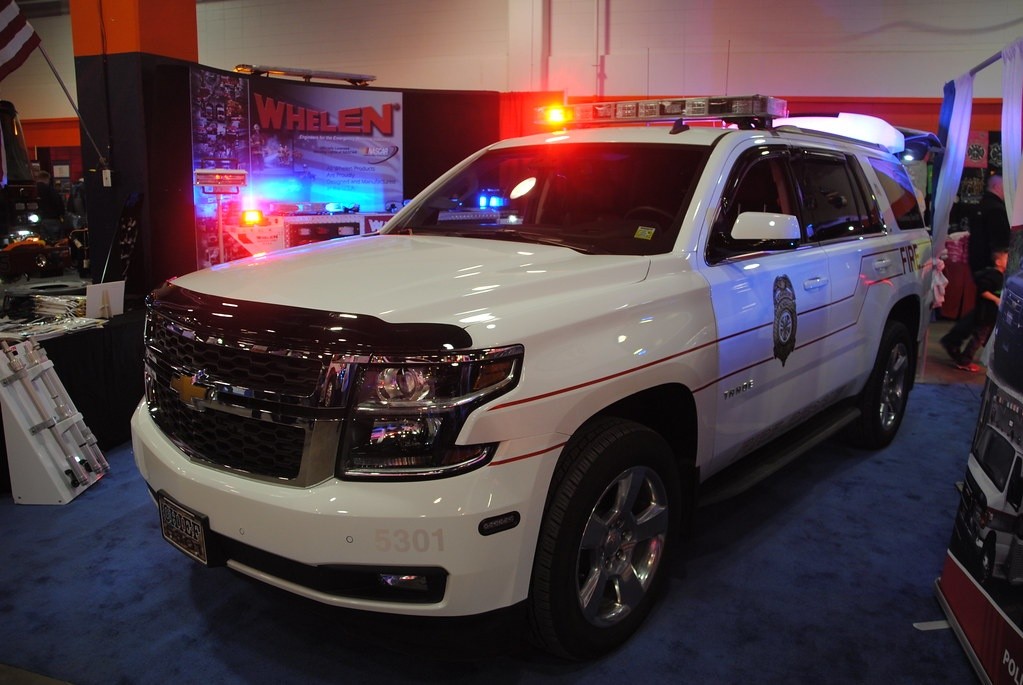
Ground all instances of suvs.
[130,120,934,665]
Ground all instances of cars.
[0,228,71,278]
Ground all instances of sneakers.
[956,357,982,372]
[940,334,961,360]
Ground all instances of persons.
[938,170,1012,373]
[36,171,91,279]
